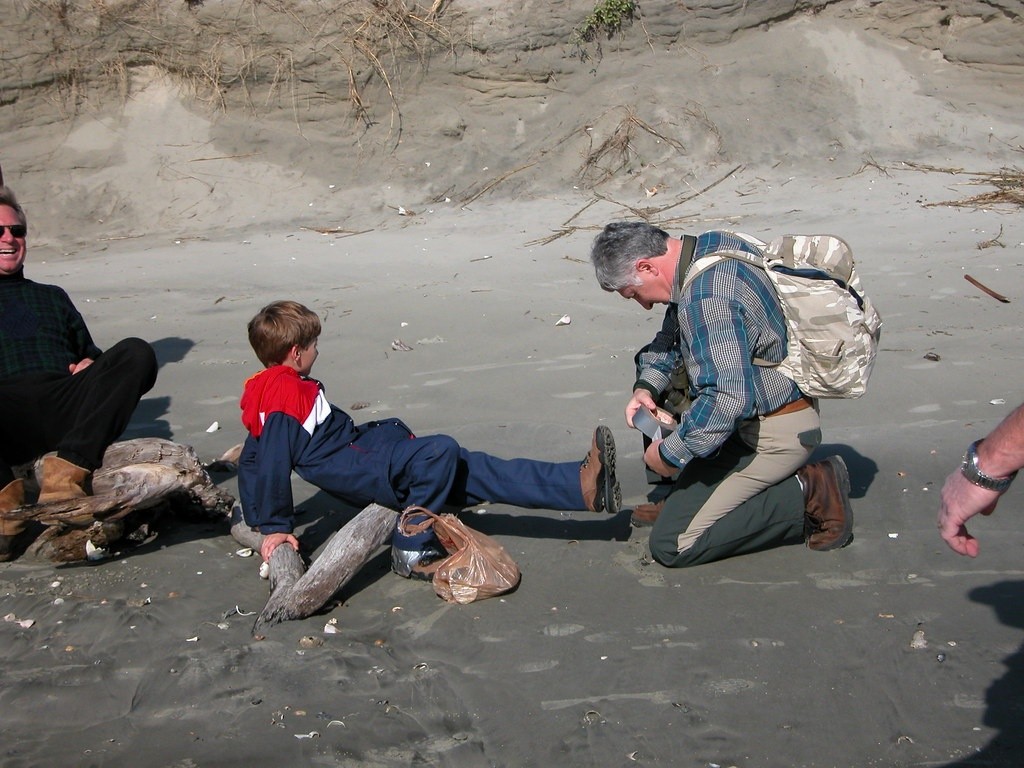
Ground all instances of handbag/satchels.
[399,504,521,606]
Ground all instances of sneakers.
[630,503,664,526]
[391,546,452,581]
[575,426,622,513]
[794,454,852,550]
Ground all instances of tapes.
[630,404,678,441]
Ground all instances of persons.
[591,222,853,569]
[238,300,622,582]
[0,186,158,562]
[938,403,1024,558]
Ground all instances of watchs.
[961,438,1017,491]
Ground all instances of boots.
[37,456,95,526]
[0,478,30,561]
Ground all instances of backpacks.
[678,229,881,400]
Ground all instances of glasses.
[0,226,26,237]
